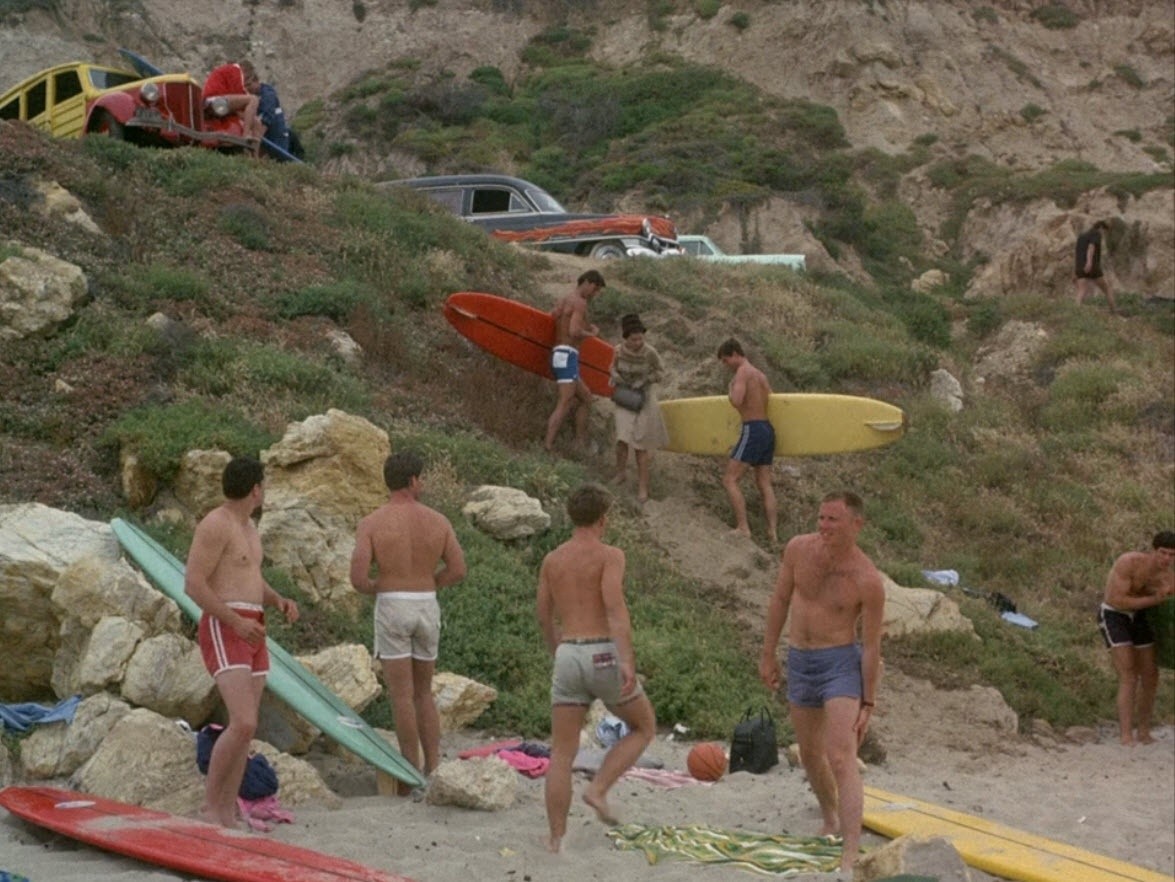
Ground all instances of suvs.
[368,173,681,265]
[0,61,205,149]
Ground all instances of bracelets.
[862,701,874,706]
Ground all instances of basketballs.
[687,742,726,781]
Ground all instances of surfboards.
[861,790,1173,881]
[657,394,904,454]
[444,294,617,397]
[109,518,427,788]
[0,787,419,882]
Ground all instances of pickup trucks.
[618,236,812,276]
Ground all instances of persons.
[1075,220,1128,321]
[543,268,605,454]
[183,456,301,830]
[349,451,466,777]
[242,72,289,161]
[608,313,665,501]
[535,484,657,851]
[718,338,779,543]
[203,60,262,155]
[1097,530,1175,746]
[759,491,886,871]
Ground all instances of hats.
[622,314,646,338]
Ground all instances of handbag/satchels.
[616,385,644,412]
[729,708,778,774]
